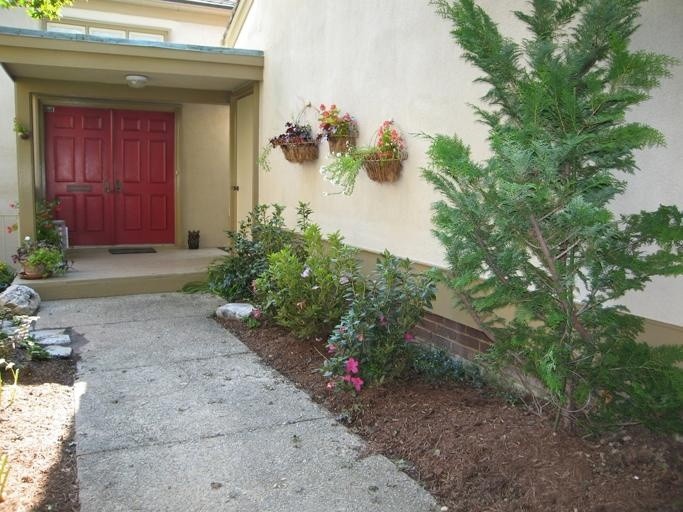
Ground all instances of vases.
[20,258,46,278]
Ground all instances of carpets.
[108,246,156,255]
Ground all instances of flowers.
[12,237,51,262]
[255,99,406,197]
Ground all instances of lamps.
[126,76,147,89]
